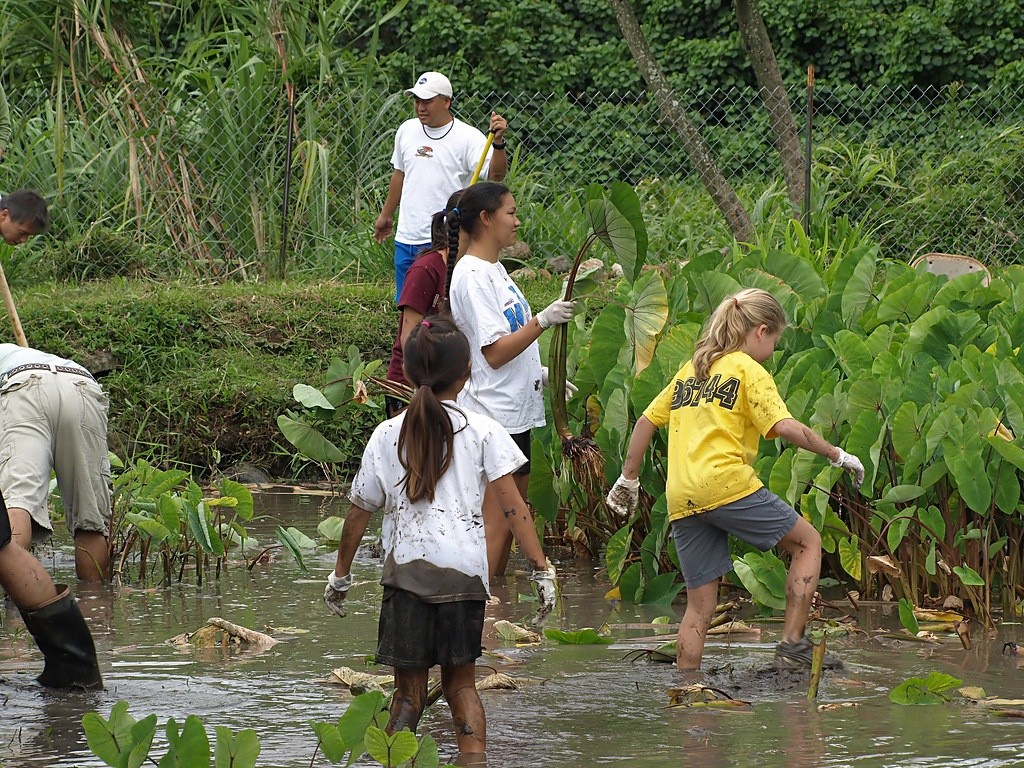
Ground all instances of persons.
[0,191,115,694]
[442,181,579,584]
[605,288,867,665]
[373,71,508,417]
[321,316,558,753]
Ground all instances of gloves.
[542,366,578,401]
[827,447,865,489]
[605,474,640,517]
[536,299,579,329]
[530,567,557,623]
[322,570,351,618]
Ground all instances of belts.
[1,363,97,383]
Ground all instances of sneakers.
[774,637,843,674]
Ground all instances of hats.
[404,72,454,100]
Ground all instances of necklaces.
[422,110,454,140]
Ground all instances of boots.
[20,584,103,692]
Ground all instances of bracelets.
[492,138,507,150]
